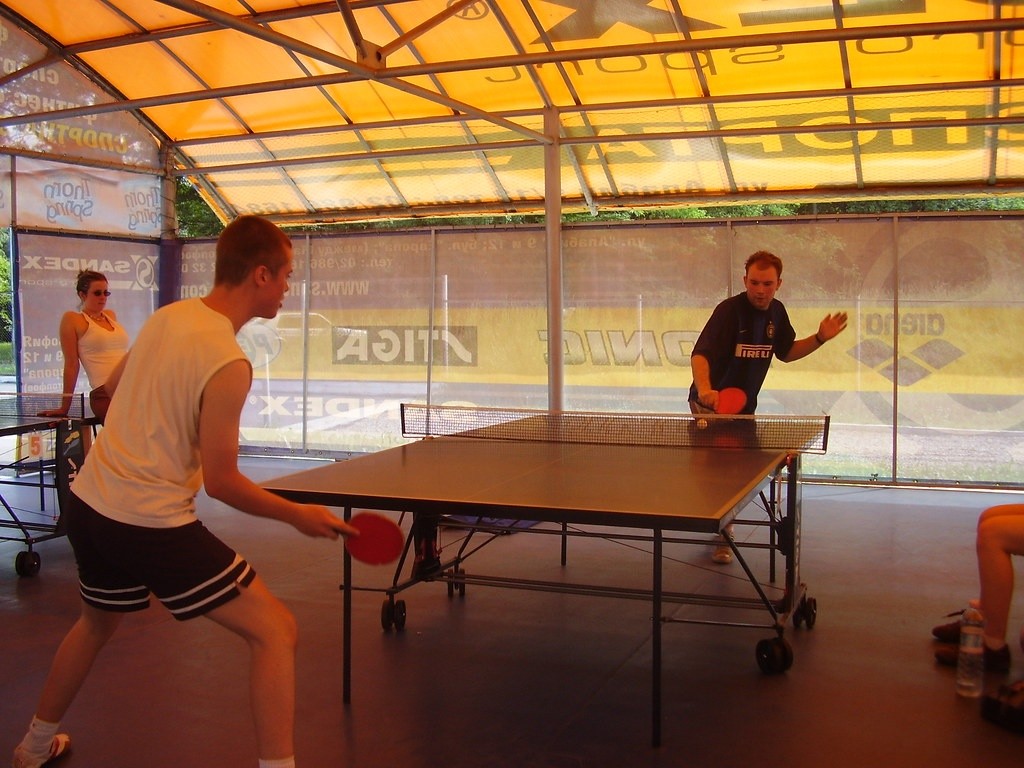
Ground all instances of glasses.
[88,291,111,296]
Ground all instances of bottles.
[956,599,988,698]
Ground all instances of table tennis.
[697,419,708,430]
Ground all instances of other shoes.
[931,620,963,641]
[935,643,1010,674]
[13,733,70,768]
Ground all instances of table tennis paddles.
[696,387,748,414]
[342,510,406,566]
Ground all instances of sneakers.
[713,534,734,562]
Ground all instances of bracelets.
[815,332,826,345]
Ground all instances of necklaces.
[81,309,107,322]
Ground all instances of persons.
[931,504,1024,671]
[687,250,849,563]
[36,269,128,418]
[981,679,1024,736]
[8,217,360,768]
